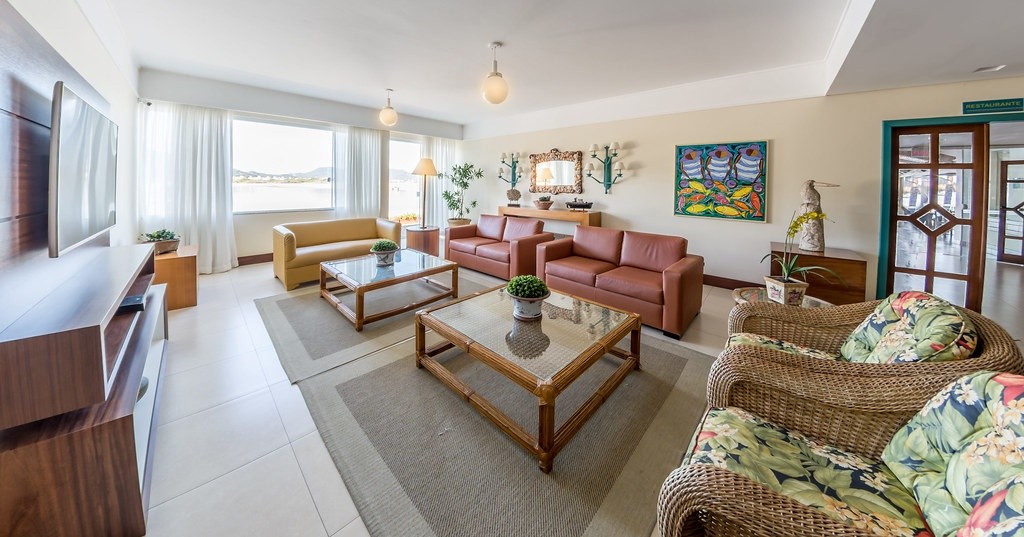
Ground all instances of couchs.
[536,224,706,341]
[272,216,402,291]
[444,213,555,281]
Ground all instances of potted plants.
[370,238,400,267]
[436,161,485,226]
[504,317,550,360]
[136,227,182,256]
[505,274,551,322]
[532,195,554,210]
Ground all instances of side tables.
[731,287,836,306]
[405,224,439,257]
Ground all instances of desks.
[498,204,602,227]
[770,241,868,306]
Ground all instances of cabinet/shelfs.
[0,243,170,537]
[152,244,198,311]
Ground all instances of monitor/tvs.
[48,81,119,258]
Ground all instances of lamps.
[497,151,523,188]
[538,167,555,185]
[497,287,512,302]
[480,40,509,105]
[411,157,438,229]
[583,141,626,194]
[581,302,623,342]
[380,88,398,126]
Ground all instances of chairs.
[657,354,1024,537]
[705,288,1024,377]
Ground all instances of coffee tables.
[414,283,642,475]
[319,246,459,332]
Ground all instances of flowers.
[760,209,849,289]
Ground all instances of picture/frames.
[673,140,769,224]
[528,148,583,195]
[541,291,584,325]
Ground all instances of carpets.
[295,329,718,537]
[253,270,490,386]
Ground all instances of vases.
[763,275,809,306]
